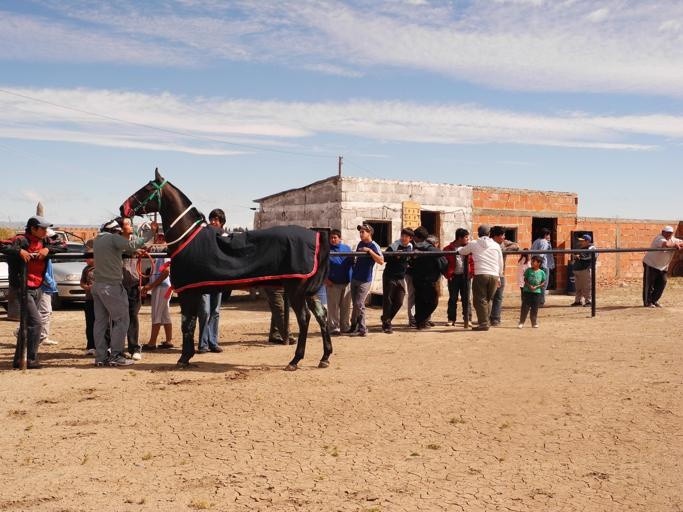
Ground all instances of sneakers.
[655,301,663,307]
[28,361,44,367]
[43,339,57,345]
[449,320,541,330]
[583,302,592,306]
[571,302,581,306]
[645,303,655,307]
[87,343,173,367]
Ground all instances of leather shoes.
[268,335,283,343]
[211,347,222,352]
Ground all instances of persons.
[139,233,173,350]
[196,209,230,353]
[13,227,59,345]
[642,226,683,309]
[114,217,168,359]
[267,224,556,345]
[91,219,158,368]
[571,234,599,307]
[80,247,114,356]
[0,215,53,368]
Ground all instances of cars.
[0,231,91,313]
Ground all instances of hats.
[106,220,123,232]
[578,234,592,241]
[27,216,53,227]
[357,224,373,233]
[663,226,673,232]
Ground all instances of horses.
[118,166,334,372]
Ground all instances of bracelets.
[368,248,372,253]
